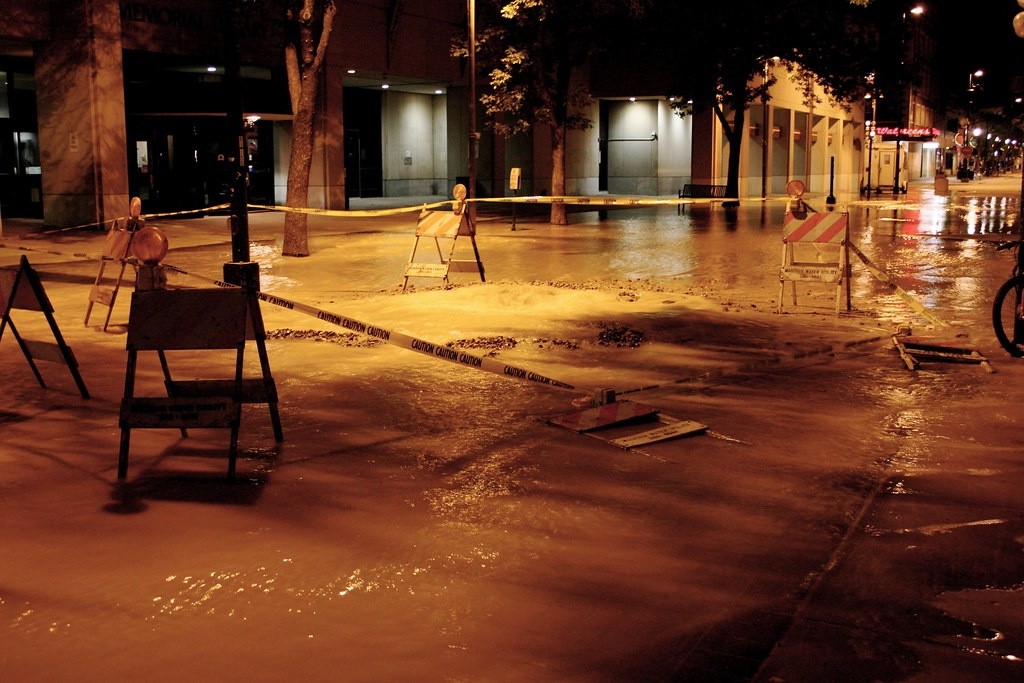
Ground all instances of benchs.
[678,184,727,198]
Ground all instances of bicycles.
[991,240,1024,358]
